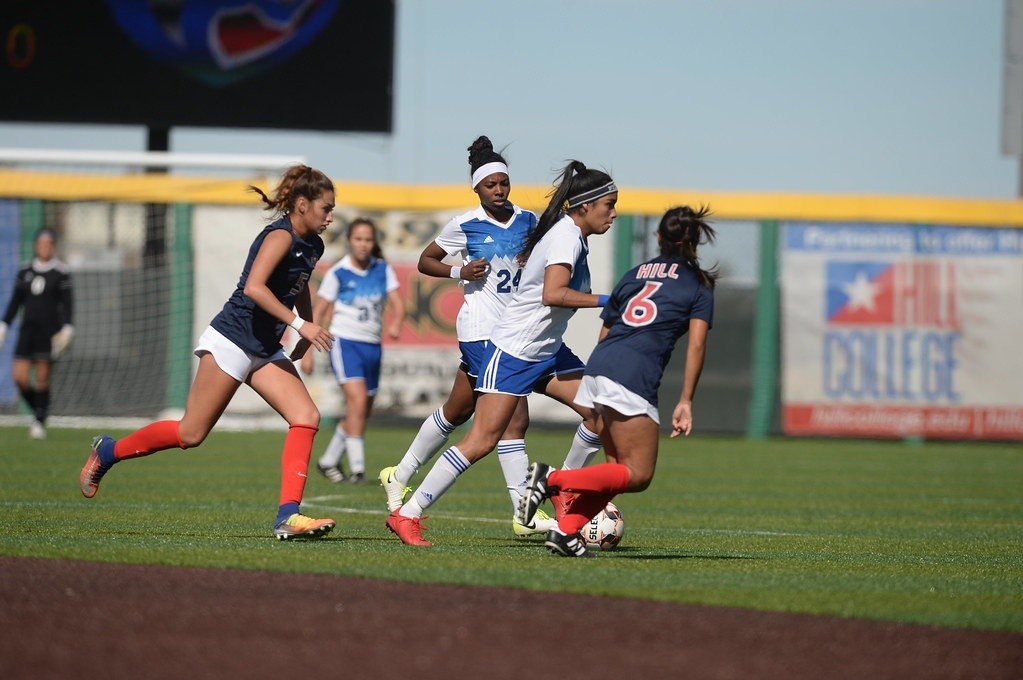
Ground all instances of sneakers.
[81,434,113,498]
[274,514,336,540]
[512,509,559,536]
[349,472,366,483]
[317,463,347,483]
[383,506,432,547]
[518,462,560,525]
[378,467,412,512]
[543,526,595,558]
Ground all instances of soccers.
[579,501,624,549]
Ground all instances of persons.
[80,165,336,540]
[303,218,406,482]
[378,135,563,538]
[385,160,618,548]
[512,205,724,558]
[0,229,77,440]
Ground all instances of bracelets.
[291,316,305,332]
[598,294,610,308]
[450,266,463,280]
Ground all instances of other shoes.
[31,420,48,439]
